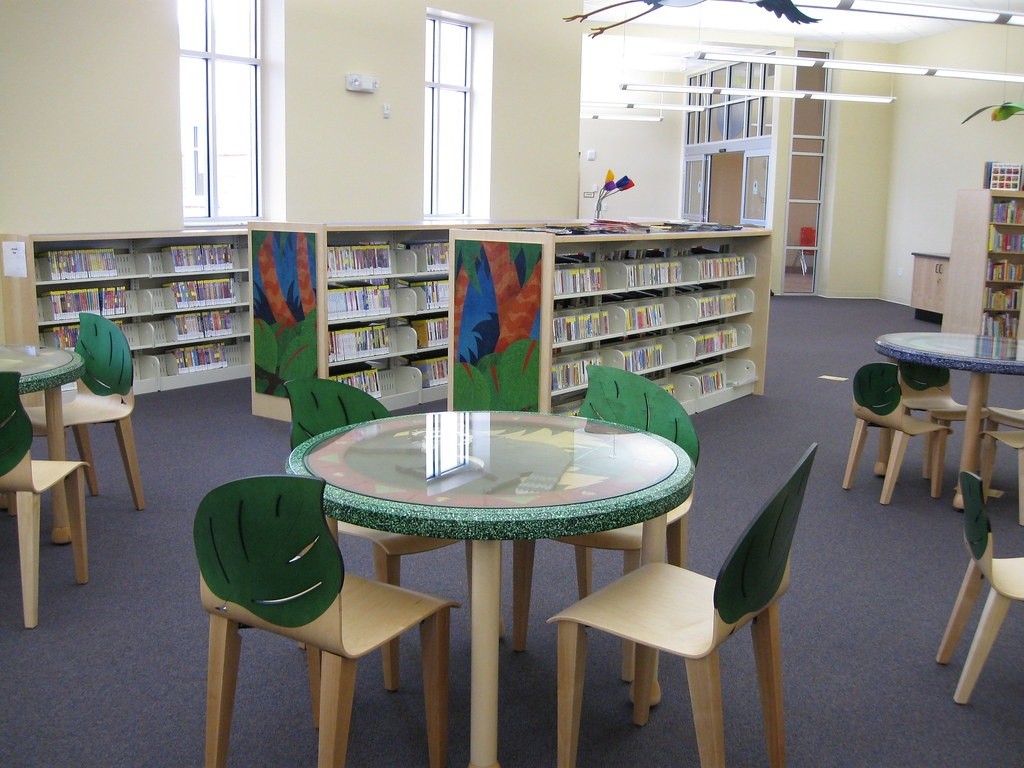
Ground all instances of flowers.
[596,169,635,203]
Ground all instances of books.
[464,218,743,234]
[38,246,126,356]
[550,245,747,424]
[325,240,450,405]
[978,198,1024,341]
[163,242,235,375]
[982,160,993,189]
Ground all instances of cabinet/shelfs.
[911,255,949,324]
[247,220,590,422]
[0,230,252,395]
[447,227,772,416]
[940,188,1024,339]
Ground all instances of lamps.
[580,114,664,122]
[580,71,705,112]
[691,19,1024,82]
[792,0,1024,26]
[619,11,896,104]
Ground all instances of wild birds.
[560,0,820,40]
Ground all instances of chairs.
[193,474,462,768]
[25,312,146,511]
[545,442,820,768]
[986,406,1024,431]
[898,361,990,479]
[0,371,92,628]
[791,227,815,277]
[283,378,506,690]
[977,431,1024,526]
[842,362,954,505]
[934,472,1024,703]
[511,365,700,681]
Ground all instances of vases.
[594,204,603,219]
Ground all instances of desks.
[874,331,1024,513]
[0,342,85,544]
[285,408,696,768]
